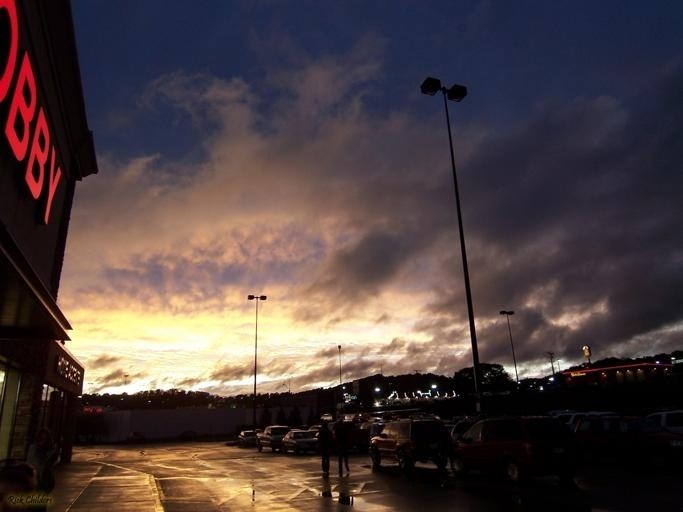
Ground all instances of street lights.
[247,295,267,430]
[421,76,487,415]
[500,310,519,382]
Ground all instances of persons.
[319,418,350,473]
